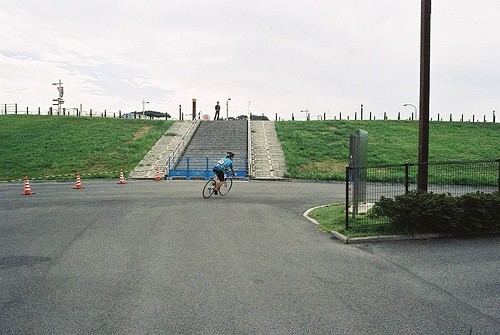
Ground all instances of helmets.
[227,152,235,157]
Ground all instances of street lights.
[403,103,417,120]
[52,79,64,116]
[226,97,231,120]
[301,109,310,121]
[142,100,149,119]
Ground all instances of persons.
[214,102,220,121]
[213,151,237,195]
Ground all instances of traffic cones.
[117,167,127,184]
[153,166,161,181]
[72,171,84,190]
[21,175,35,196]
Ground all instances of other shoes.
[213,189,218,195]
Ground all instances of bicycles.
[202,171,233,199]
